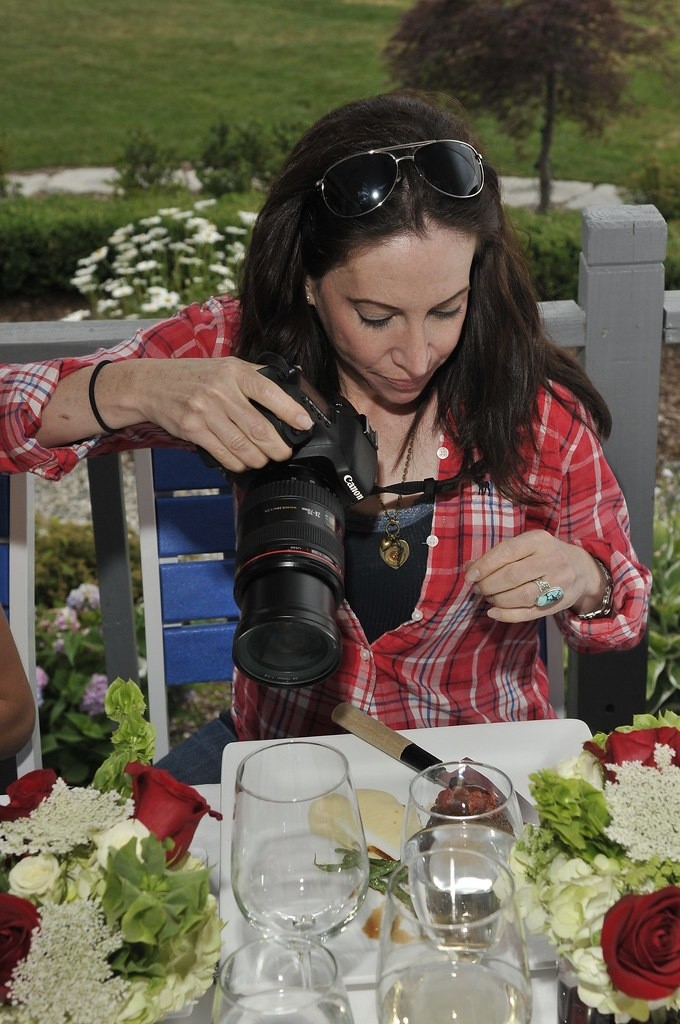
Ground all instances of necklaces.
[373,427,417,570]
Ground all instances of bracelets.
[88,359,121,434]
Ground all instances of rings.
[532,578,563,608]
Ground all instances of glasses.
[309,139,485,218]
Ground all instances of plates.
[219,719,605,996]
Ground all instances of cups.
[209,938,354,1024]
[375,849,534,1024]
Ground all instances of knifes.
[330,703,543,828]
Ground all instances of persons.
[0,95,653,787]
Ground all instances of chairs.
[86,442,244,764]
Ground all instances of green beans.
[311,848,417,913]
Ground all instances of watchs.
[577,558,615,620]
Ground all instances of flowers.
[0,674,230,1024]
[492,707,680,1023]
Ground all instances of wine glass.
[230,742,371,991]
[400,762,525,967]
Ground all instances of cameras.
[196,363,378,689]
